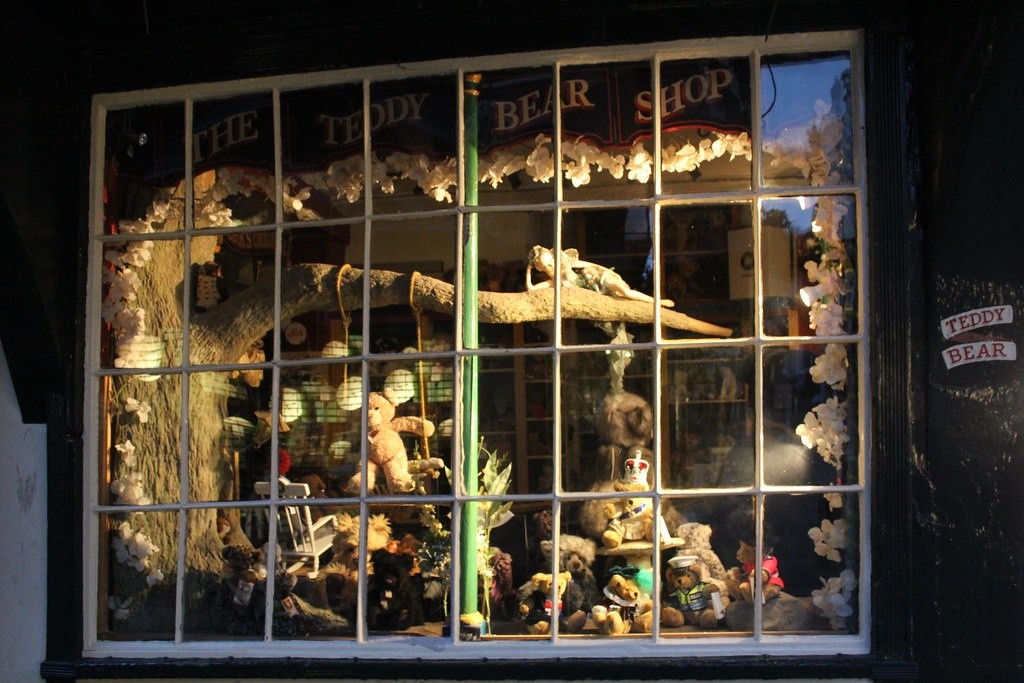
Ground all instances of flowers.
[104,99,856,629]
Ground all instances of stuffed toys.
[490,483,786,642]
[229,510,445,634]
[344,389,436,497]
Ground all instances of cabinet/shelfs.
[576,207,752,534]
[758,221,815,353]
[331,256,571,554]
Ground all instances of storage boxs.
[728,226,792,302]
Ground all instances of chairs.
[254,475,339,576]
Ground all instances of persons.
[523,247,676,308]
